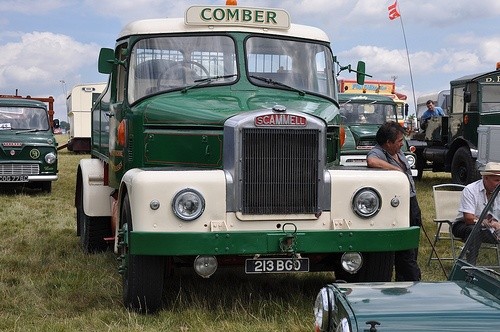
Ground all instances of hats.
[480,161,500,176]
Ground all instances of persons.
[421,99,446,120]
[365,120,423,282]
[449,161,500,268]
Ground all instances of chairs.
[427,184,500,270]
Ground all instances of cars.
[53,128,62,135]
[337,61,500,186]
[0,88,61,194]
[73,0,422,316]
[62,129,67,134]
[312,181,500,331]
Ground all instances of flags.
[388,0,401,21]
